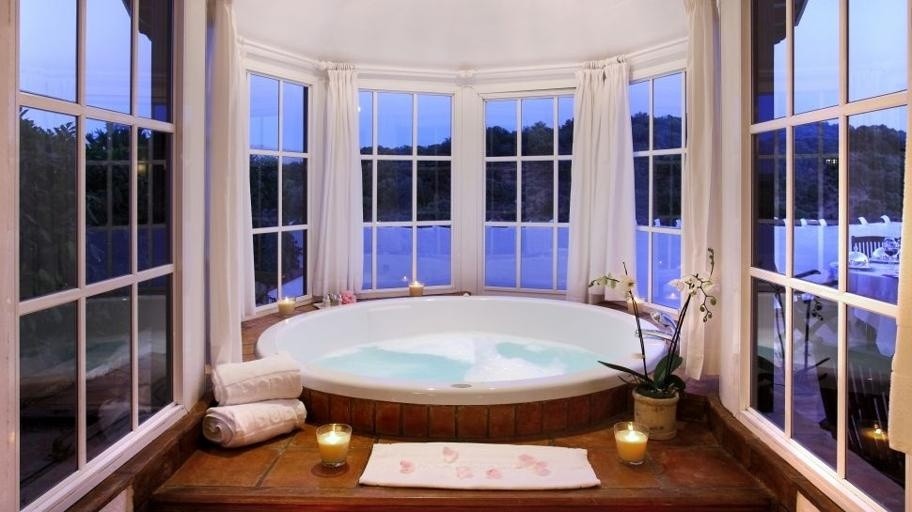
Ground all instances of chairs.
[813,340,905,490]
[850,234,886,262]
[773,267,844,375]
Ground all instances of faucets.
[645,308,681,356]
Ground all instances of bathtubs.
[253,293,669,407]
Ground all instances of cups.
[627,297,645,315]
[277,296,295,318]
[316,422,350,467]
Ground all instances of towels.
[201,398,307,448]
[210,355,304,407]
[359,441,603,492]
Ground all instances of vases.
[630,386,681,444]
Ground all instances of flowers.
[585,243,728,397]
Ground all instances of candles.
[313,420,353,473]
[277,296,298,317]
